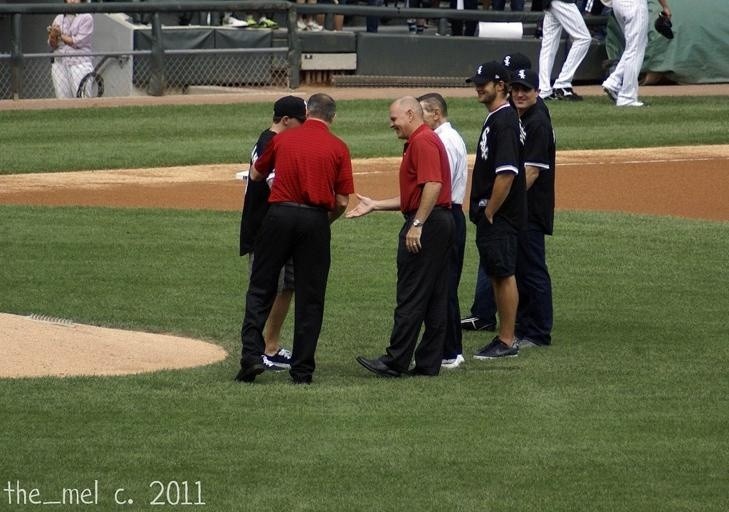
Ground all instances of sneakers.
[261,346,292,371]
[440,314,537,370]
[545,87,651,106]
[223,15,323,32]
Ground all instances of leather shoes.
[357,355,402,378]
[407,368,438,376]
[234,357,264,383]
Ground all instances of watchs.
[412,219,423,228]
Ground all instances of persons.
[460,53,532,329]
[601,1,671,106]
[47,0,96,99]
[469,61,528,360]
[236,93,354,383]
[132,1,538,33]
[346,96,455,376]
[417,93,469,368]
[240,95,307,369]
[537,0,592,100]
[509,68,555,351]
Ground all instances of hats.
[466,52,539,90]
[655,12,673,39]
[273,96,308,115]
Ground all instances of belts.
[279,202,316,208]
